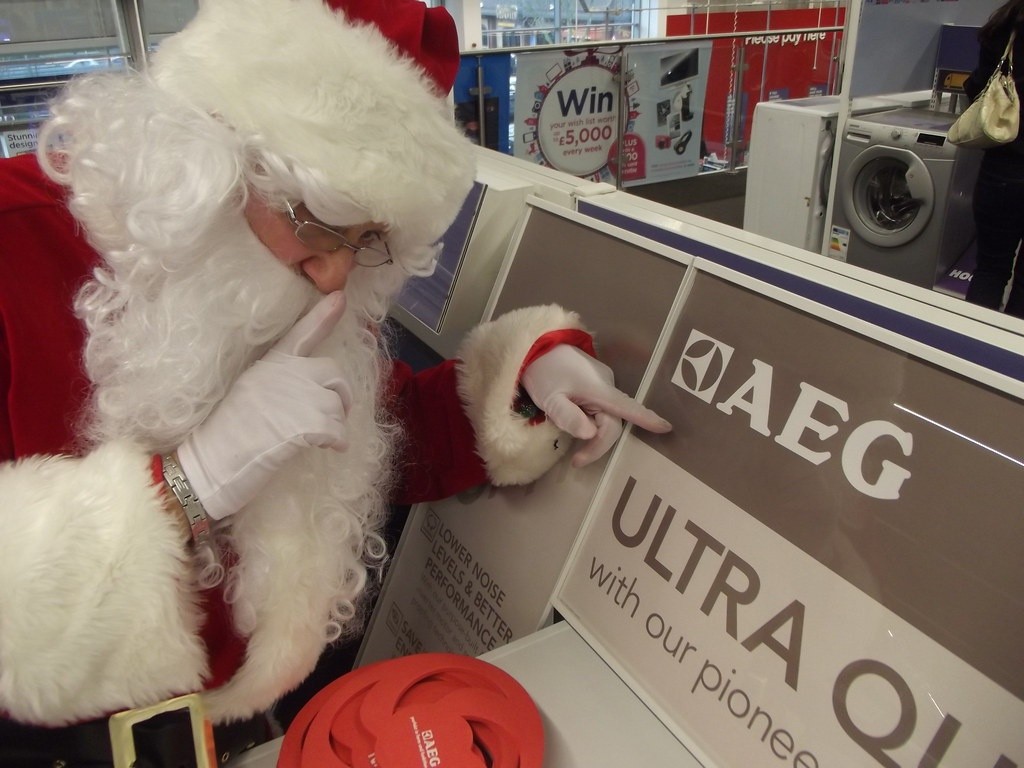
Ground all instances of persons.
[2,0,676,732]
[960,0,1024,316]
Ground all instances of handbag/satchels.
[944,30,1020,155]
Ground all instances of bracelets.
[161,451,214,554]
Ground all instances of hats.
[152,1,482,250]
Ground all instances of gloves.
[178,290,354,520]
[523,340,673,468]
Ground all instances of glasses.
[268,175,394,268]
